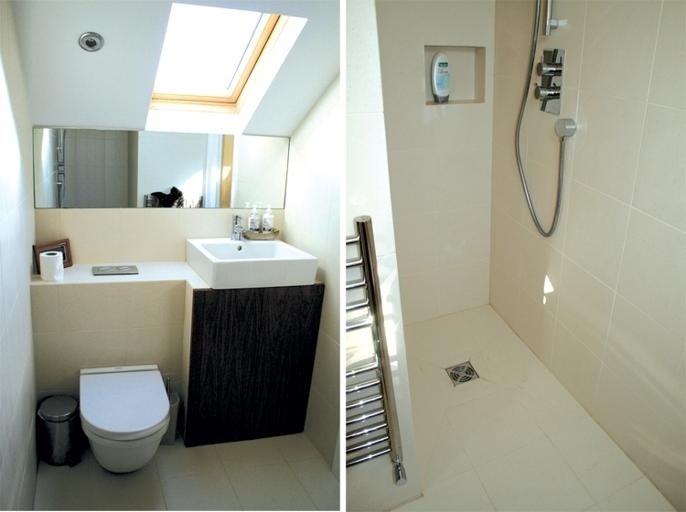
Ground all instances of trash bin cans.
[38,394,82,465]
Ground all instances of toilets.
[77,365,170,474]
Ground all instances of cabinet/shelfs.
[31,262,326,448]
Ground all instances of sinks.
[185,236,320,288]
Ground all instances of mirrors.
[33,127,292,211]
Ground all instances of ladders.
[347,215,405,487]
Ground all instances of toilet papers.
[39,250,65,281]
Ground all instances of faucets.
[232,214,245,239]
